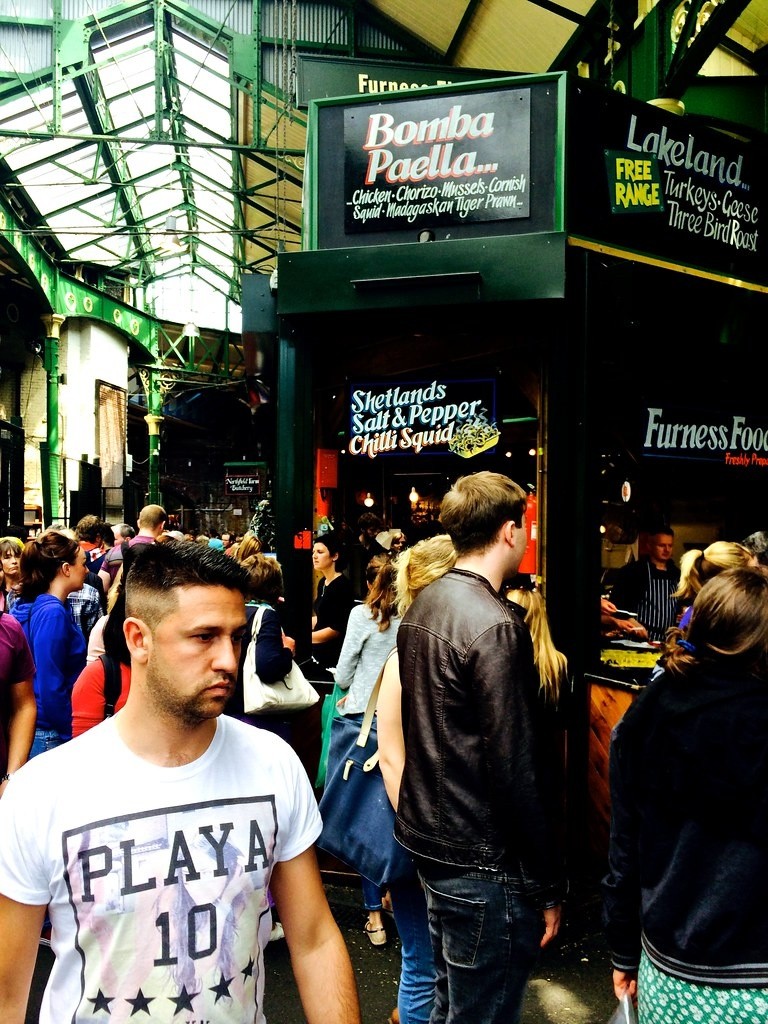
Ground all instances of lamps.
[417,228,437,242]
[181,320,200,337]
[165,144,177,232]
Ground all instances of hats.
[207,538,223,551]
[162,530,184,541]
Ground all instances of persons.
[2,498,354,941]
[0,539,361,1023]
[590,519,768,1024]
[312,463,591,1024]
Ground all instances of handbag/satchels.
[242,603,320,714]
[313,711,400,886]
[316,682,352,788]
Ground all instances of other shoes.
[382,898,395,918]
[364,921,386,945]
